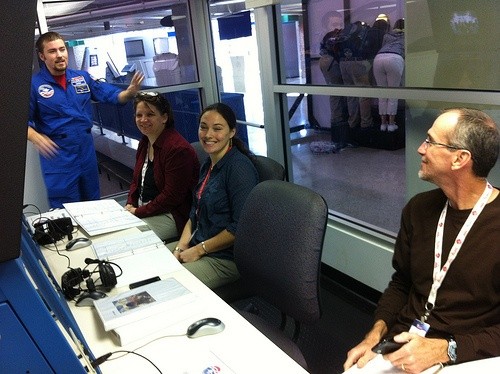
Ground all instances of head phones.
[62,263,116,298]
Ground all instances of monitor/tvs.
[125,36,148,60]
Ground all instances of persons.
[28,32,144,211]
[166,103,259,291]
[320,27,345,131]
[340,20,391,130]
[373,18,405,133]
[124,90,201,240]
[341,107,500,374]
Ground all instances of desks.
[27,209,310,374]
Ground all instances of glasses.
[424,136,475,162]
[134,91,166,105]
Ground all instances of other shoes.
[380,124,388,131]
[388,124,398,132]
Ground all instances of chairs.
[248,154,285,182]
[232,180,329,371]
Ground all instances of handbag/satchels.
[311,141,337,153]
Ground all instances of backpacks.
[319,28,345,60]
[352,26,379,56]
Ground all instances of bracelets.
[202,241,208,255]
[175,247,183,253]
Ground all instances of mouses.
[75,290,109,306]
[186,318,225,339]
[66,238,92,251]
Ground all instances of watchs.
[446,335,458,365]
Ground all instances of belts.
[340,57,367,61]
